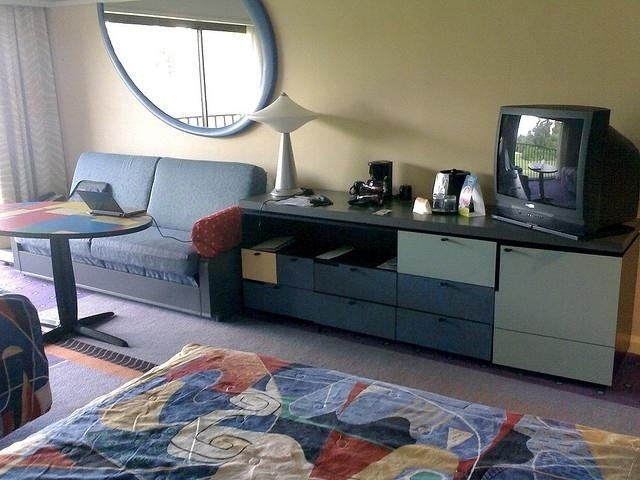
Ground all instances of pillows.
[192,206,242,257]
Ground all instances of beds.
[0,341,640,480]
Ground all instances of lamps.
[243,91,322,202]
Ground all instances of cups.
[444,195,456,212]
[432,193,445,212]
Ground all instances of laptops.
[76,189,147,218]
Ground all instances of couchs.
[7,148,273,321]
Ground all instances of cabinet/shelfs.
[236,185,640,398]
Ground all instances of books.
[251,236,296,252]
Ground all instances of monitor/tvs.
[491,105,640,241]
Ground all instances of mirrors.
[96,0,281,137]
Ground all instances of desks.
[0,201,154,353]
